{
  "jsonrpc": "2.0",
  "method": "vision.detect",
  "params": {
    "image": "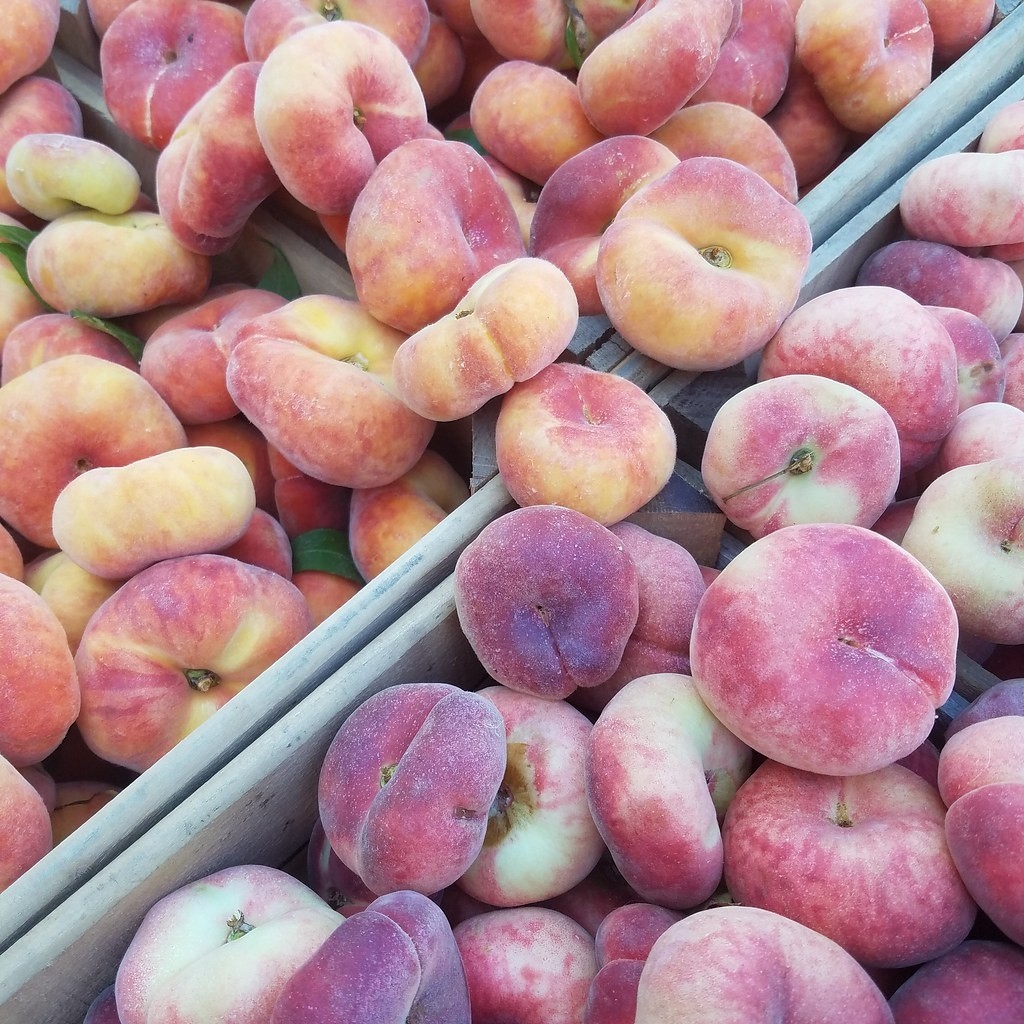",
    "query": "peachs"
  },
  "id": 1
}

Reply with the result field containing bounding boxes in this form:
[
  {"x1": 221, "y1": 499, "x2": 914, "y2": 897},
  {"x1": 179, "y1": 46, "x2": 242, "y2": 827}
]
[{"x1": 0, "y1": 0, "x2": 1024, "y2": 1024}]
